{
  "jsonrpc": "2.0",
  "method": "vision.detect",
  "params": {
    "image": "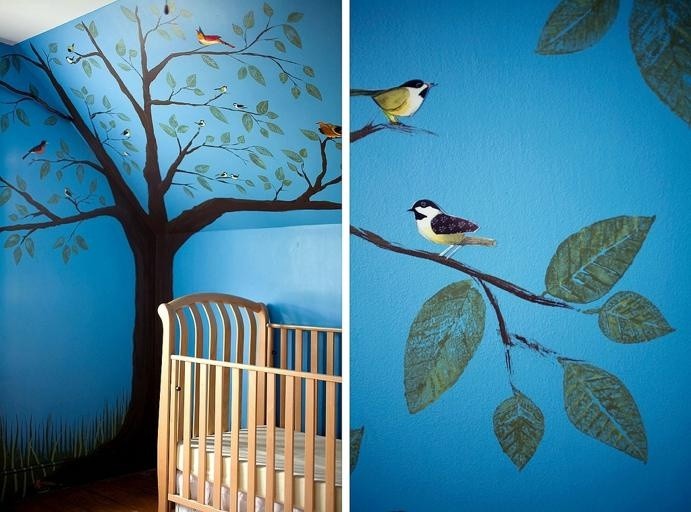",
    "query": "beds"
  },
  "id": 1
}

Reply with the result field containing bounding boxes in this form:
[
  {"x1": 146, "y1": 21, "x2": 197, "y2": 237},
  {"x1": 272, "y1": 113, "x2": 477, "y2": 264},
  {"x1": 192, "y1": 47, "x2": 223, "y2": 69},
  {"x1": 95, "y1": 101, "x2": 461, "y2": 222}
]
[{"x1": 157, "y1": 293, "x2": 342, "y2": 512}]
